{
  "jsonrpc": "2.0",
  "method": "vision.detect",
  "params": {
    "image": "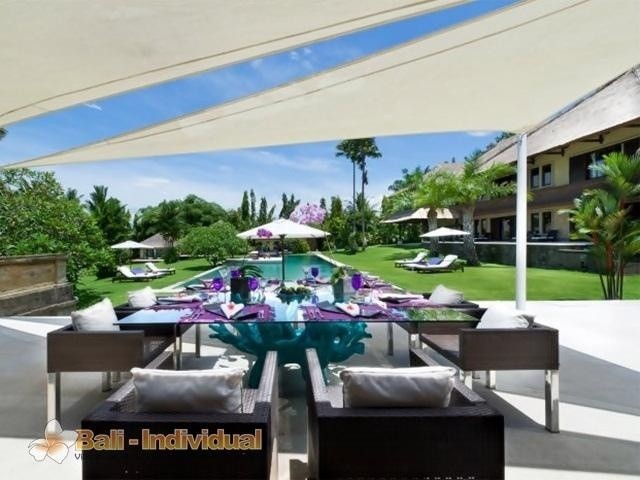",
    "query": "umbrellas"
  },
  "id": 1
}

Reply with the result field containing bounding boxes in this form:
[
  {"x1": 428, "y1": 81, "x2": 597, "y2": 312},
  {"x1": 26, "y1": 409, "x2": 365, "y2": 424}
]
[
  {"x1": 417, "y1": 226, "x2": 472, "y2": 257},
  {"x1": 235, "y1": 216, "x2": 332, "y2": 281},
  {"x1": 108, "y1": 239, "x2": 153, "y2": 270}
]
